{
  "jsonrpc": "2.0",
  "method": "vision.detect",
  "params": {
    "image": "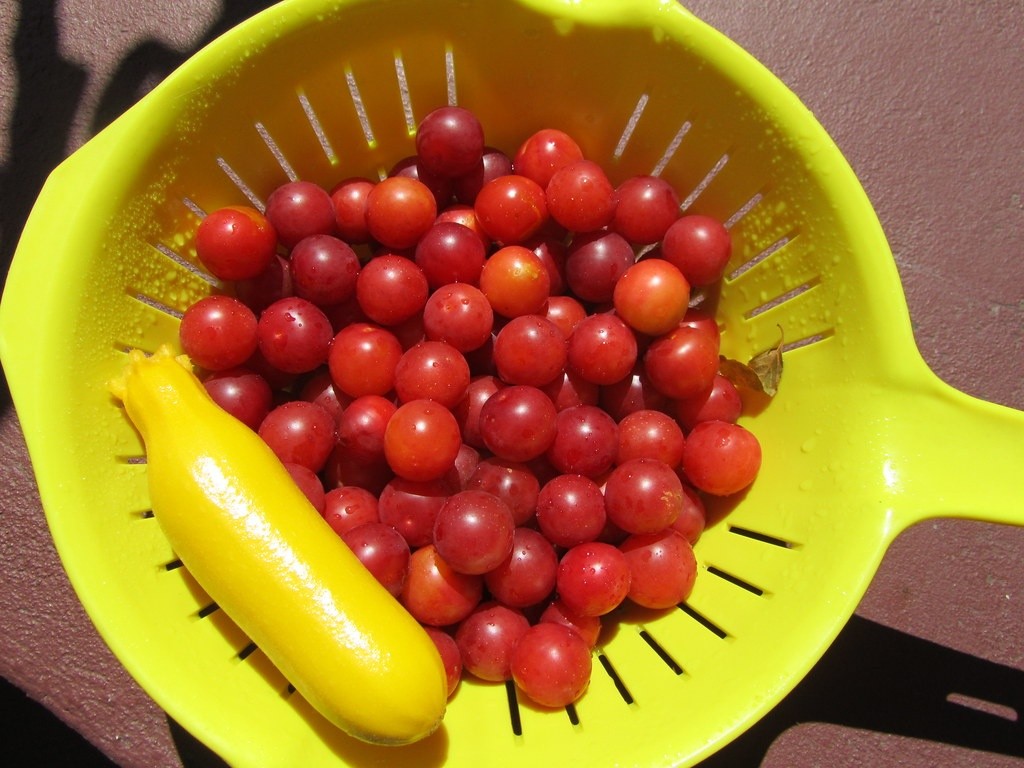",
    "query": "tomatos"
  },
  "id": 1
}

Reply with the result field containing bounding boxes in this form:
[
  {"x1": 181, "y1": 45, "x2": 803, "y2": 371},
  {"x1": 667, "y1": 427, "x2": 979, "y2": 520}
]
[{"x1": 180, "y1": 108, "x2": 762, "y2": 707}]
